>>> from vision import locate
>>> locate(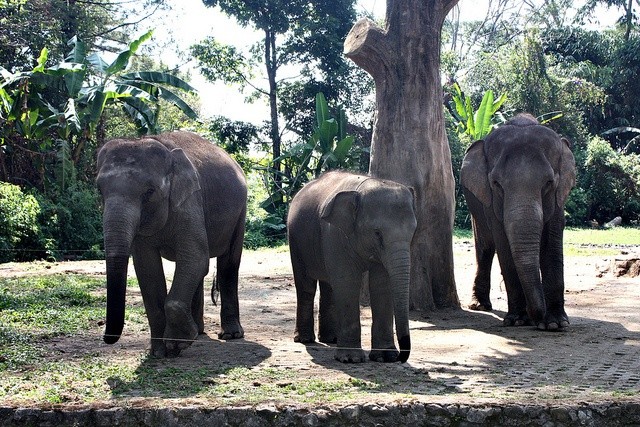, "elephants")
[94,127,248,360]
[285,167,419,366]
[459,113,576,333]
